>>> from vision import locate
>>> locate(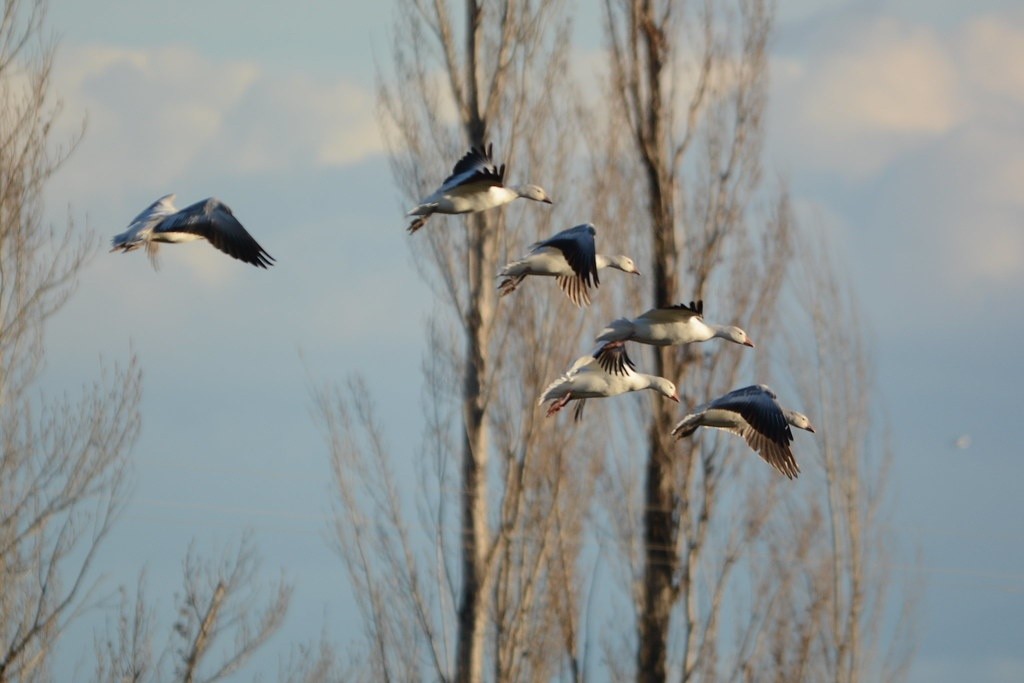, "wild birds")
[108,192,278,272]
[404,141,817,481]
